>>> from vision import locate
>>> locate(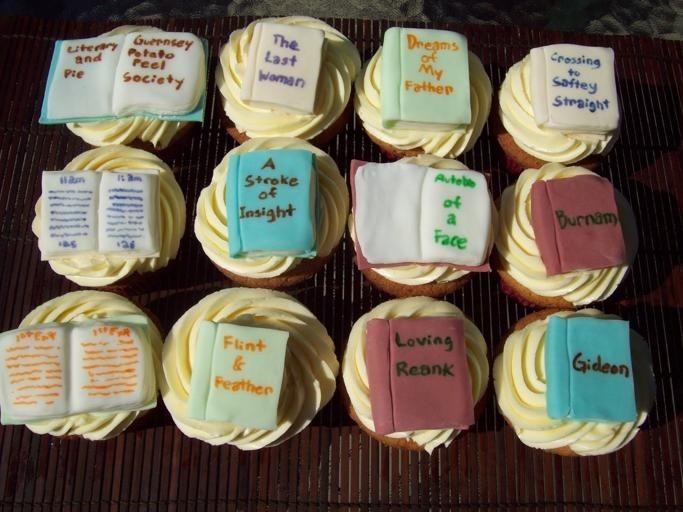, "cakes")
[493,163,640,308]
[497,43,622,166]
[214,14,361,145]
[159,289,340,453]
[193,136,348,288]
[33,143,186,288]
[348,160,498,298]
[0,291,163,442]
[339,294,492,457]
[352,26,494,158]
[37,25,208,151]
[490,308,658,458]
